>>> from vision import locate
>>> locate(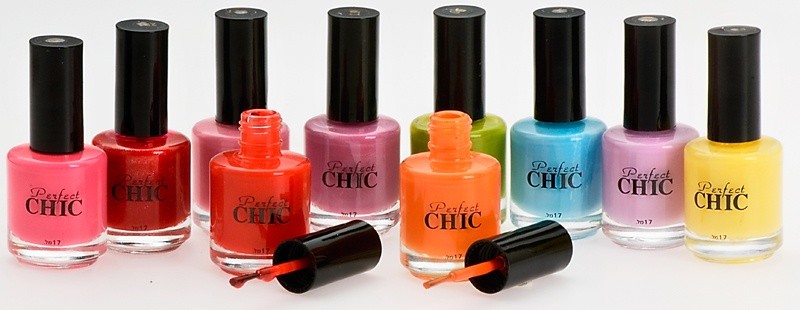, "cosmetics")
[5,5,783,294]
